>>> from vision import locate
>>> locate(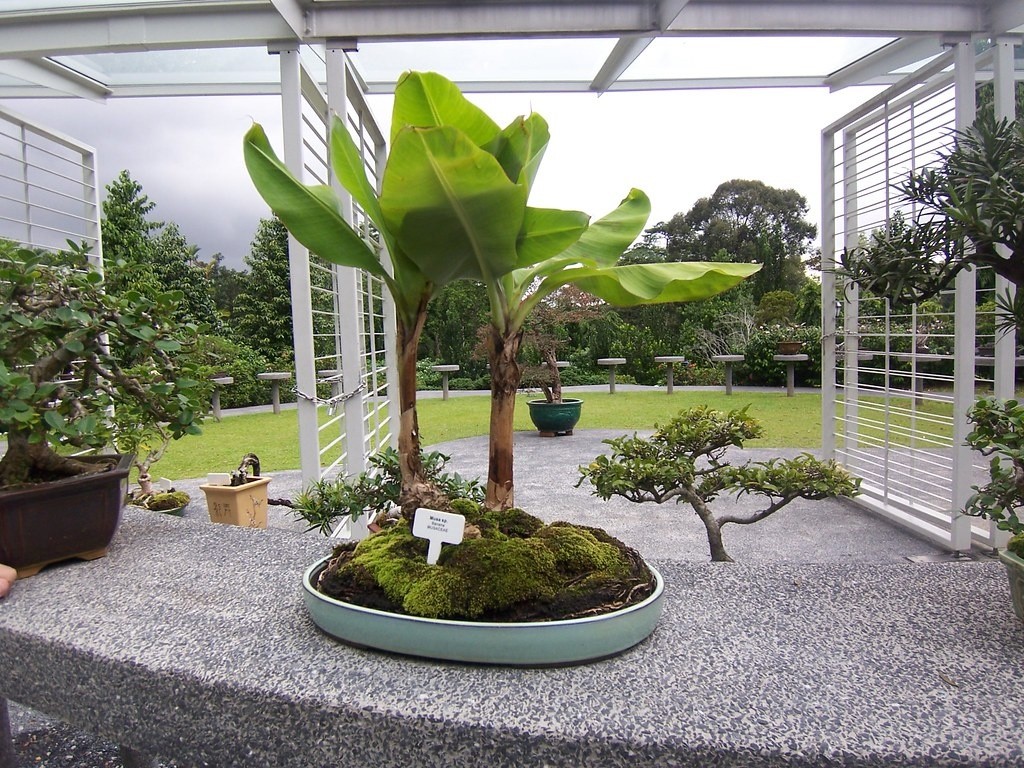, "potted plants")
[0,238,212,580]
[114,418,191,517]
[776,317,806,355]
[473,284,605,432]
[810,104,1024,623]
[243,70,764,661]
[199,452,358,537]
